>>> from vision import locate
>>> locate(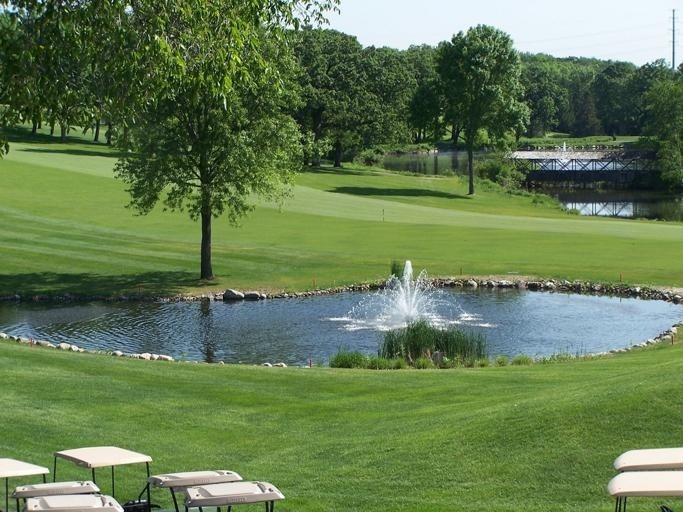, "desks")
[613,447,683,473]
[0,445,285,512]
[607,471,683,512]
